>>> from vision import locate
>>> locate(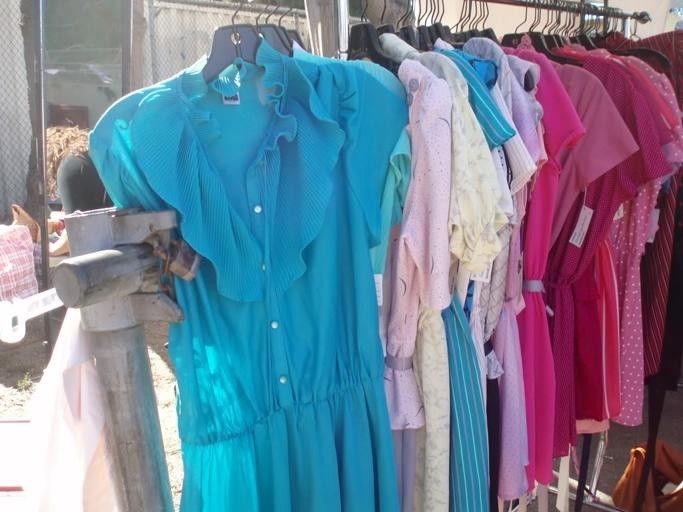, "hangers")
[169,1,682,100]
